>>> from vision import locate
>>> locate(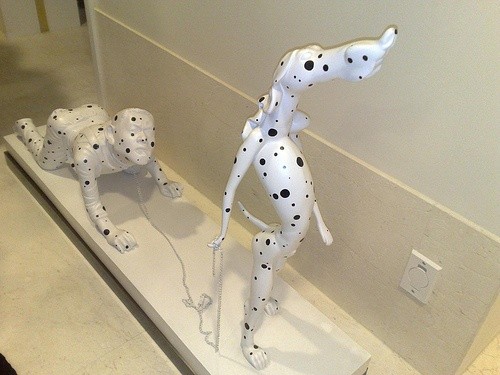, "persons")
[13,102,184,255]
